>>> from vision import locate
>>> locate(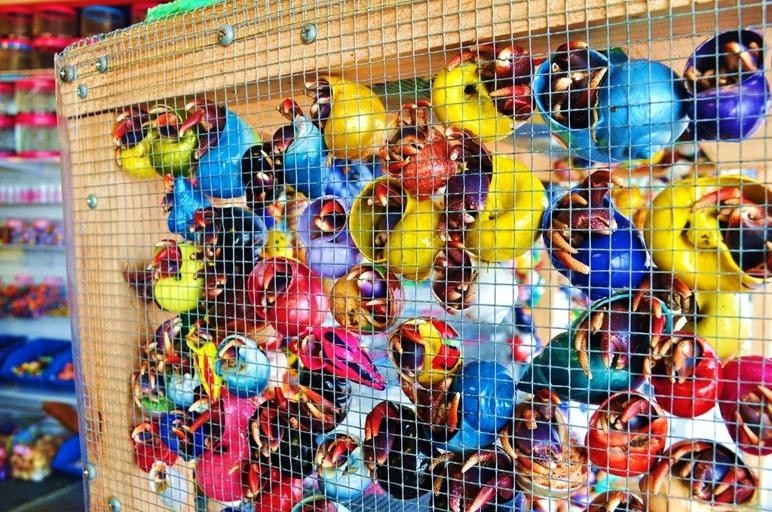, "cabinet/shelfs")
[0,0,100,512]
